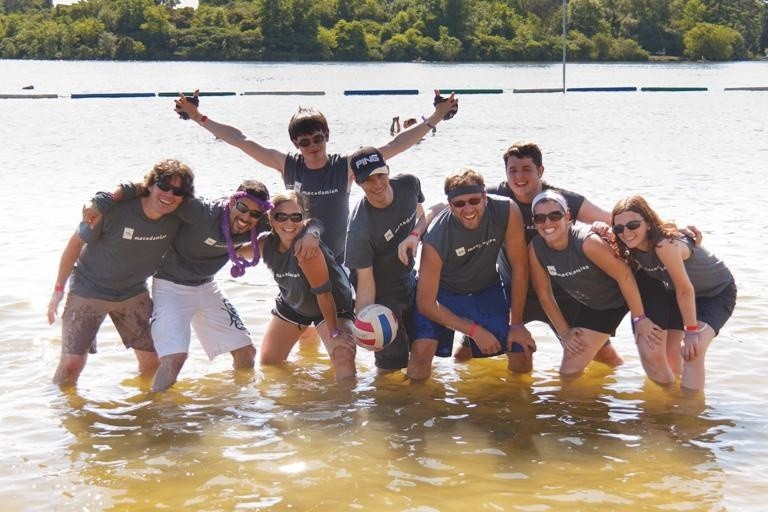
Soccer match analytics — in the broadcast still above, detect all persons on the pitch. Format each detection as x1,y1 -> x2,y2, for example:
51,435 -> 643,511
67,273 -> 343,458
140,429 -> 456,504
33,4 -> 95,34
396,143 -> 702,367
342,144 -> 426,372
80,181 -> 326,394
173,89 -> 459,340
403,170 -> 537,381
523,189 -> 674,388
48,160 -> 195,384
229,189 -> 358,385
591,196 -> 738,392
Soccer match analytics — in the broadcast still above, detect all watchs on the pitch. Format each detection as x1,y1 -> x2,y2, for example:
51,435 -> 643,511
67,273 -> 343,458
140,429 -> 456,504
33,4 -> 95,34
304,228 -> 321,241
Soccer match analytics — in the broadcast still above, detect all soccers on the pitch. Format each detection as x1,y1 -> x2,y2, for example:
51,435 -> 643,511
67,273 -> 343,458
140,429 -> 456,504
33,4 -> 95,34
352,303 -> 399,350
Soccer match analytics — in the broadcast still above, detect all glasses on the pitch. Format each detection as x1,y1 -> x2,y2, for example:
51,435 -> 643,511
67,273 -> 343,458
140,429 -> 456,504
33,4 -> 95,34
156,180 -> 185,196
612,218 -> 646,233
452,194 -> 484,206
533,212 -> 565,224
274,213 -> 303,222
298,134 -> 326,146
236,200 -> 263,217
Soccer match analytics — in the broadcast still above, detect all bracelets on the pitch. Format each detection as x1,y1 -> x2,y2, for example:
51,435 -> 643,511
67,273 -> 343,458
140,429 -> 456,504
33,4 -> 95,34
55,285 -> 64,292
199,115 -> 208,126
510,322 -> 524,331
466,321 -> 478,337
632,314 -> 647,324
408,231 -> 422,241
421,116 -> 434,129
328,327 -> 344,338
683,325 -> 698,332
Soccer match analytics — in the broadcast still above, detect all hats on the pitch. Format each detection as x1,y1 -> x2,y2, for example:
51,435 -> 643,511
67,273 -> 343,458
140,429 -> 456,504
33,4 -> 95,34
350,146 -> 389,181
